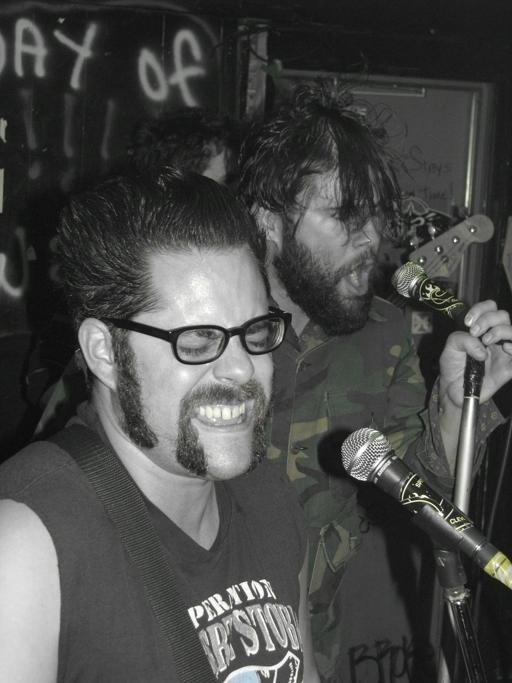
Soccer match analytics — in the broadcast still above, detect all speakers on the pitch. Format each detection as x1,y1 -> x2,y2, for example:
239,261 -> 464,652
266,67 -> 503,314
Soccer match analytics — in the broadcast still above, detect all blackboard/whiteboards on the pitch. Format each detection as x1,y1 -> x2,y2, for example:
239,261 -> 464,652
0,2 -> 242,459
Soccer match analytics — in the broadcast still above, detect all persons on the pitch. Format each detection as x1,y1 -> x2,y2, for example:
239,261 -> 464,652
0,163 -> 323,682
230,76 -> 511,683
29,104 -> 258,442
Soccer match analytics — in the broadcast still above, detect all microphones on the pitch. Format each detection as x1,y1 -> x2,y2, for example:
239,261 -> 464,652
389,260 -> 504,346
340,426 -> 512,592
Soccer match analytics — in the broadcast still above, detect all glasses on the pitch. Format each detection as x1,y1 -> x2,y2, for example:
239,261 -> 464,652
102,303 -> 293,366
